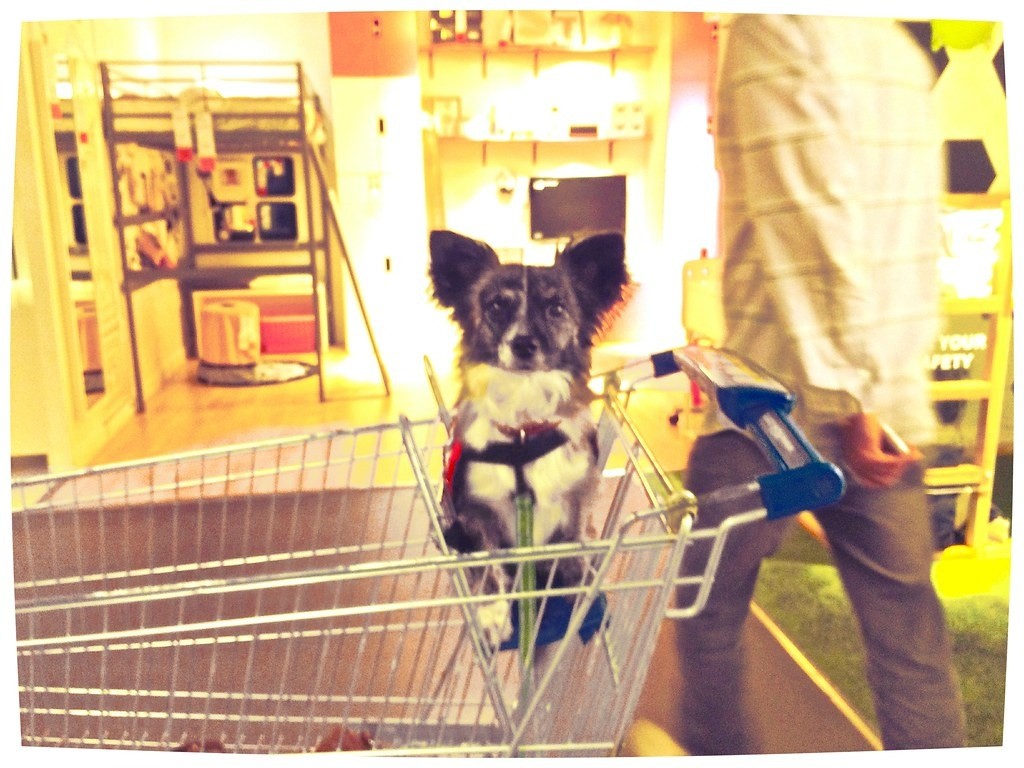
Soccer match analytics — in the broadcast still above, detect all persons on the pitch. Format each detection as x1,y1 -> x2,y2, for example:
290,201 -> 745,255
673,11 -> 968,757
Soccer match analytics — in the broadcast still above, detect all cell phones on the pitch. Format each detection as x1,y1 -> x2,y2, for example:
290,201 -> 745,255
879,422 -> 910,456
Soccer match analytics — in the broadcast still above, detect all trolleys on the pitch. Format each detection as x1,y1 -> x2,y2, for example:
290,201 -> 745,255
12,342 -> 852,758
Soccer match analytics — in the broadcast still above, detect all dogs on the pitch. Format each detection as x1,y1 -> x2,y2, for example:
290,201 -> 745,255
426,224 -> 631,661
